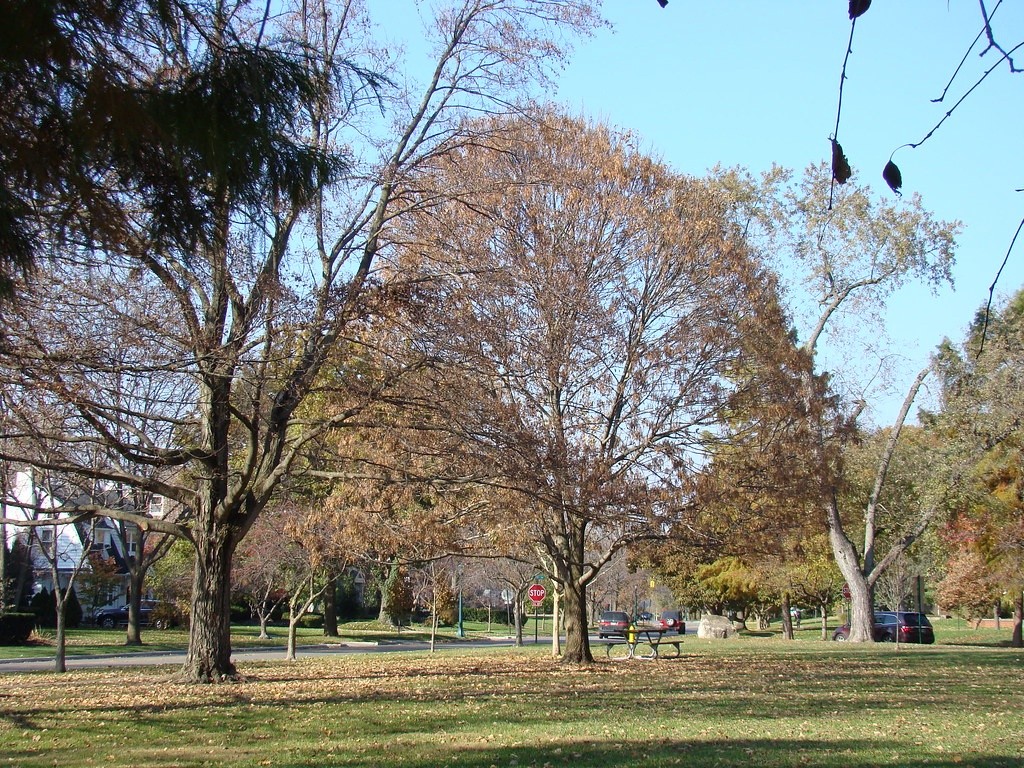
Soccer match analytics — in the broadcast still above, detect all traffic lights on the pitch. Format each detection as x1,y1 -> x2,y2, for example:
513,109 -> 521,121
650,579 -> 656,588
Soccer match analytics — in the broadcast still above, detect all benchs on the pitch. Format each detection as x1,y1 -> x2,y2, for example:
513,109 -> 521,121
642,640 -> 684,646
603,642 -> 643,645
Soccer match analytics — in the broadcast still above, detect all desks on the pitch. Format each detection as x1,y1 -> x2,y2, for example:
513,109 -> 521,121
606,629 -> 680,661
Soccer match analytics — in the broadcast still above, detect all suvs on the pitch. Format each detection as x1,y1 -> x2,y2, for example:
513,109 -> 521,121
659,610 -> 685,634
95,600 -> 184,630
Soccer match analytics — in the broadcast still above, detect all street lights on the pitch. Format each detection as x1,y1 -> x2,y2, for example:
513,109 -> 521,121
634,584 -> 638,625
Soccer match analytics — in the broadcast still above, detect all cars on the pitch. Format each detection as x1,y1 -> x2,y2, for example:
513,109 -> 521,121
642,614 -> 650,620
831,611 -> 935,644
598,611 -> 635,639
790,608 -> 799,616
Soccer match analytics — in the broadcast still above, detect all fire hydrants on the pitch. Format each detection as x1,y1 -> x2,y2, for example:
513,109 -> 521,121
629,626 -> 635,644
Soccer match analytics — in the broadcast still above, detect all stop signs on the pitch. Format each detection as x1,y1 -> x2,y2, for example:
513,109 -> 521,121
842,582 -> 852,599
528,584 -> 546,602
